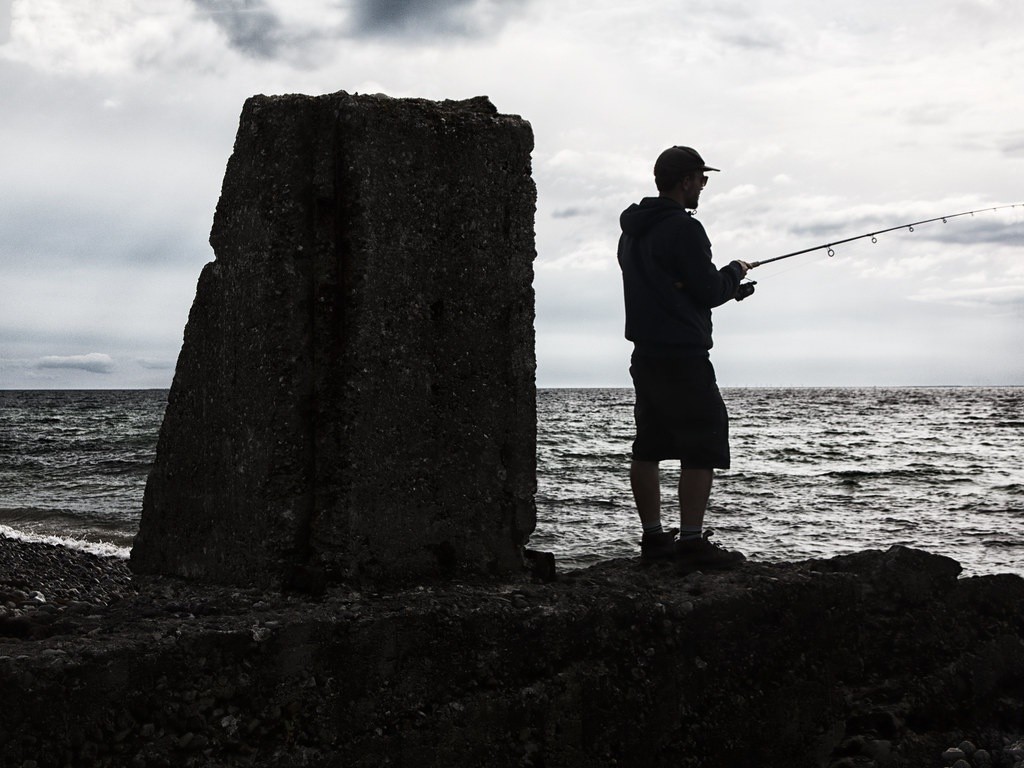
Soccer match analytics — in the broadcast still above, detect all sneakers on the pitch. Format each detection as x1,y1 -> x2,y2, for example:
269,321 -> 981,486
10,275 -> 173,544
673,530 -> 746,569
638,526 -> 680,561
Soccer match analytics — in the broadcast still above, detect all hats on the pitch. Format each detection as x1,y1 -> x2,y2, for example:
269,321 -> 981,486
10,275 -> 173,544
654,146 -> 720,175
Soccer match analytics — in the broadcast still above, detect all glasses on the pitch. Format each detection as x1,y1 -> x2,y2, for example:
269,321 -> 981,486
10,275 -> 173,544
687,175 -> 708,187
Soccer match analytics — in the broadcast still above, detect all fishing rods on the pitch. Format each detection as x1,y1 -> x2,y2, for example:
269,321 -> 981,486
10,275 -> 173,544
732,203 -> 1024,302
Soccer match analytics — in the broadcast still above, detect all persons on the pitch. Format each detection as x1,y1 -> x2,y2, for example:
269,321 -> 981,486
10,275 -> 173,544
617,145 -> 757,577
